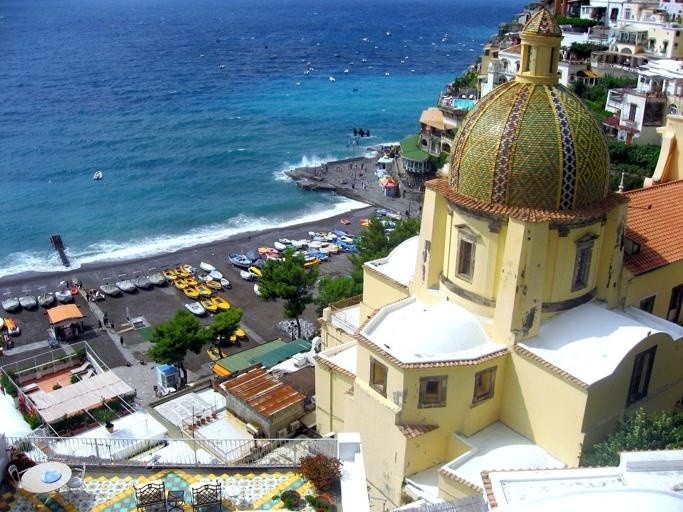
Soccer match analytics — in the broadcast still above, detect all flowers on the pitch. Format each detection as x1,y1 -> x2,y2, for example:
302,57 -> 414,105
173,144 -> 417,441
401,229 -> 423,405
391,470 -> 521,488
297,453 -> 343,488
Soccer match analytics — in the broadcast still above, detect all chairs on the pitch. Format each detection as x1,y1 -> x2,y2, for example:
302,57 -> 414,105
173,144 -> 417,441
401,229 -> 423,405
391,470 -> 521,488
80,369 -> 93,380
190,480 -> 222,512
59,463 -> 86,501
70,361 -> 90,373
133,482 -> 167,512
8,464 -> 37,504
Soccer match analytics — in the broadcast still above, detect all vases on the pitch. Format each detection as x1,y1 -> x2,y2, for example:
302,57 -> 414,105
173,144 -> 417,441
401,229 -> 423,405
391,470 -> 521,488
320,484 -> 333,491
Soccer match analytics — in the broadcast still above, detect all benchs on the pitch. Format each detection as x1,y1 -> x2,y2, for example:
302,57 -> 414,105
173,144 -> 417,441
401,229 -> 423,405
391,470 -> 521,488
21,383 -> 37,393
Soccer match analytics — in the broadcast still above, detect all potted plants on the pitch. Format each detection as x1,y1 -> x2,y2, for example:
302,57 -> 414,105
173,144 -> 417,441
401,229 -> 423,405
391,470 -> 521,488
305,493 -> 333,512
272,491 -> 301,509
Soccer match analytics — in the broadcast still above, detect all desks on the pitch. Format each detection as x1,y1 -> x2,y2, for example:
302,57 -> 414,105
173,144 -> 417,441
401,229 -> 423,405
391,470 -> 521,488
166,491 -> 185,512
21,461 -> 72,507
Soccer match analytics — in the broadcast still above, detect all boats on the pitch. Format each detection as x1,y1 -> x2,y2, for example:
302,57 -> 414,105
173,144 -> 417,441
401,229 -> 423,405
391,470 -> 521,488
2,280 -> 75,312
8,324 -> 20,337
163,261 -> 246,344
94,170 -> 102,179
341,218 -> 351,224
87,269 -> 167,301
361,208 -> 405,233
228,228 -> 360,296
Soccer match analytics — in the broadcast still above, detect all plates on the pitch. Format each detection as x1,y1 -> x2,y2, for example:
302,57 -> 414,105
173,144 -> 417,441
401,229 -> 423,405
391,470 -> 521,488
41,471 -> 62,483
